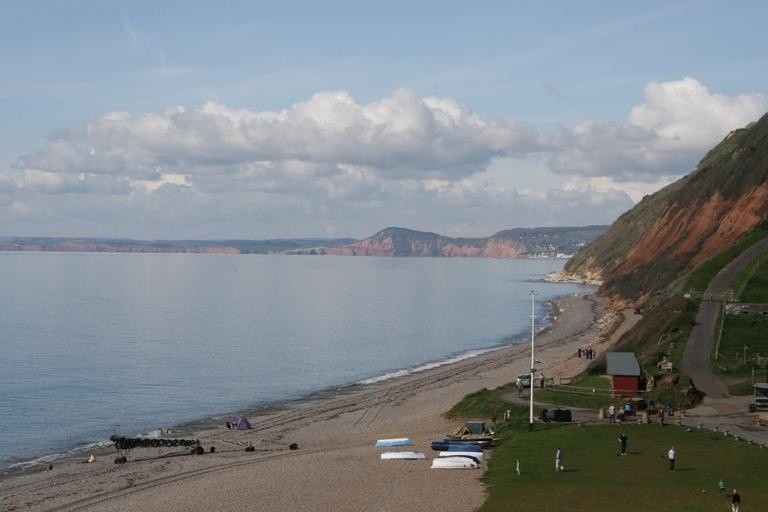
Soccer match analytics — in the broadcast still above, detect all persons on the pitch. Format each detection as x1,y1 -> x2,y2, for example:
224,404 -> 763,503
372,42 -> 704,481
578,347 -> 596,359
608,405 -> 616,423
539,372 -> 545,388
731,489 -> 740,512
719,479 -> 726,497
555,447 -> 566,473
518,381 -> 524,398
618,407 -> 625,422
668,447 -> 676,472
618,433 -> 628,456
659,408 -> 664,425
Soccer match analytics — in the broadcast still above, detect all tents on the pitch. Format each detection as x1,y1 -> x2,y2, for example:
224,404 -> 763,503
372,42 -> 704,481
237,417 -> 251,430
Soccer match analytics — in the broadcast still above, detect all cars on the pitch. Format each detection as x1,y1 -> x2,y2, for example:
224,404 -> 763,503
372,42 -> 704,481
514,374 -> 533,388
544,407 -> 571,424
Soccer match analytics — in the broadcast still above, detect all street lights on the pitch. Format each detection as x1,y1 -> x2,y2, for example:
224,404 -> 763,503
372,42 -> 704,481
528,288 -> 538,430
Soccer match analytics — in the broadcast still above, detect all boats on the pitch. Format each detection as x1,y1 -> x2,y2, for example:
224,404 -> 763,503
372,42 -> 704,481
374,435 -> 493,470
